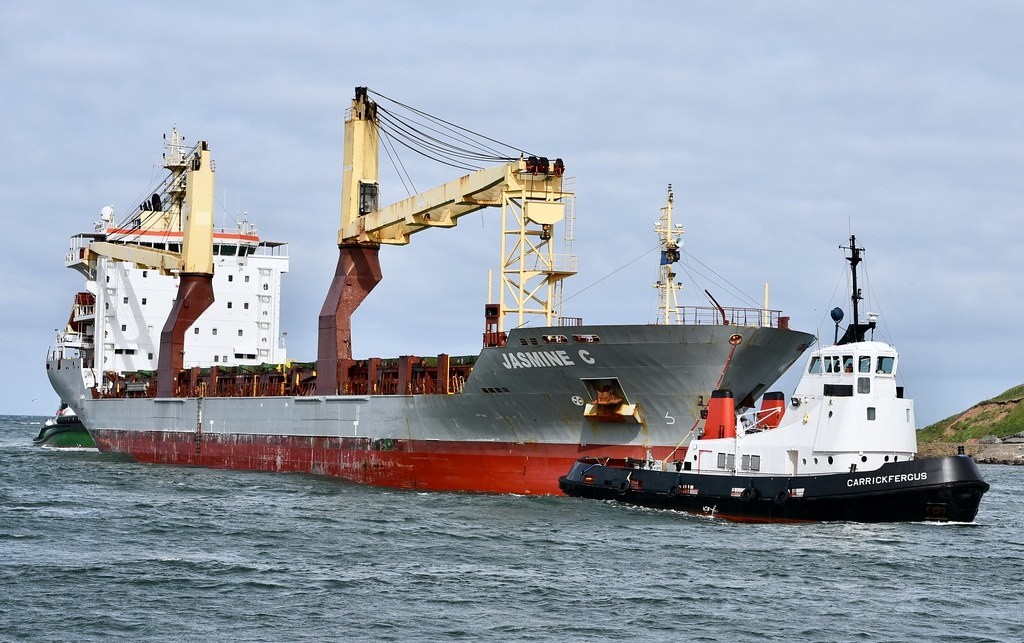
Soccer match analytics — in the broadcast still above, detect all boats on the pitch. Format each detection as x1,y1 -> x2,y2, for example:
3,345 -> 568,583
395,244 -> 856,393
556,236 -> 991,526
31,398 -> 97,449
47,83 -> 817,494
